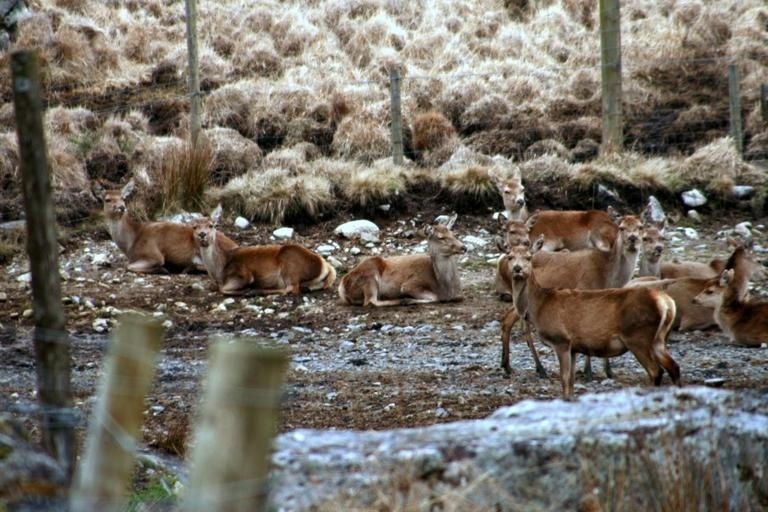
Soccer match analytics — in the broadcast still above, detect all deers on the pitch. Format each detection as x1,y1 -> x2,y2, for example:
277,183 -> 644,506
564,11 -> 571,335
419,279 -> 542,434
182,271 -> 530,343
637,216 -> 671,276
690,268 -> 768,354
337,212 -> 468,308
487,166 -> 610,248
180,203 -> 336,297
494,232 -> 681,396
499,209 -> 540,251
624,231 -> 768,332
499,205 -> 650,382
91,175 -> 240,273
